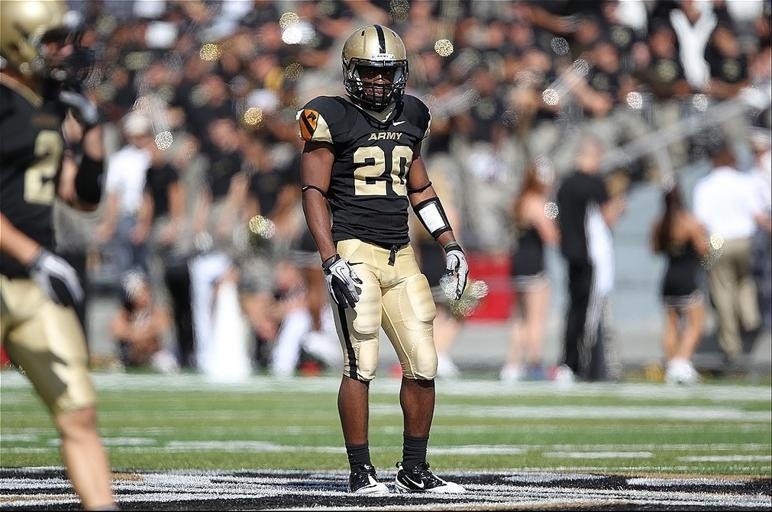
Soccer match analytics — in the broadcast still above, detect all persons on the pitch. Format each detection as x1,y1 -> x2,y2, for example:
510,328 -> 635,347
296,25 -> 468,496
2,0 -> 771,386
0,0 -> 124,512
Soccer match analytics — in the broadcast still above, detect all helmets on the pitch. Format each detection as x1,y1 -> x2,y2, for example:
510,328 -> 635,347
341,25 -> 408,108
0,1 -> 73,82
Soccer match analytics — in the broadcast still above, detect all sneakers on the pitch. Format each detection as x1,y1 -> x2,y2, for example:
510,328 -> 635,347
347,465 -> 390,497
395,462 -> 467,496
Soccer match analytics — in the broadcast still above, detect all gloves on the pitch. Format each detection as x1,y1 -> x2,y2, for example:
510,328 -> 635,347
320,253 -> 363,310
443,242 -> 470,301
30,250 -> 87,306
